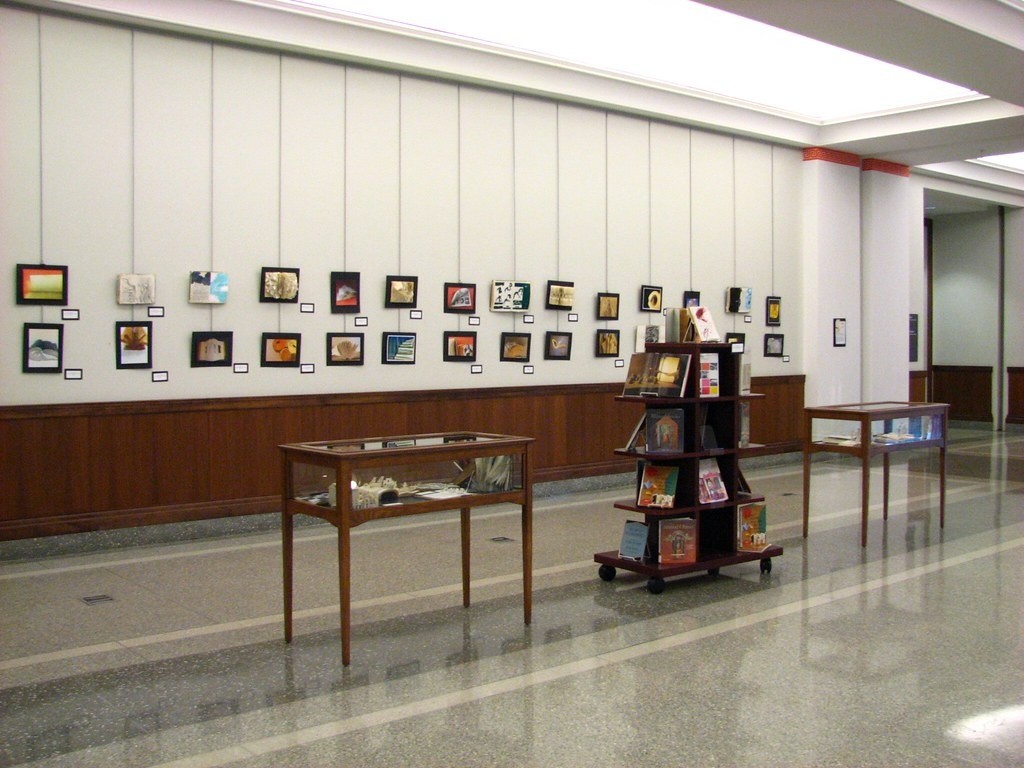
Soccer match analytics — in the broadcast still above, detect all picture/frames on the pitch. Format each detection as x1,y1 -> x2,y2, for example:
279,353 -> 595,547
189,325 -> 662,369
833,318 -> 846,347
116,267 -> 420,314
764,334 -> 784,357
16,263 -> 69,307
22,323 -> 63,374
116,320 -> 152,370
683,291 -> 700,309
765,296 -> 781,327
443,280 -> 619,320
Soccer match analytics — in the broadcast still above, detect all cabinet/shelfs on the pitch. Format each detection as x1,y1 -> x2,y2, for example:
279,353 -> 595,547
594,341 -> 784,594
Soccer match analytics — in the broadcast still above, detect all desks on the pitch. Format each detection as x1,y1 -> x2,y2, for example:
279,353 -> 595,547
276,432 -> 537,666
799,399 -> 951,548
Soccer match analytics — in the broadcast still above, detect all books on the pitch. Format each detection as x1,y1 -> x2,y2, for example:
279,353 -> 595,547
637,465 -> 679,508
700,353 -> 719,398
737,502 -> 767,548
666,307 -> 696,342
646,409 -> 684,453
698,457 -> 729,504
658,517 -> 696,563
623,352 -> 692,397
